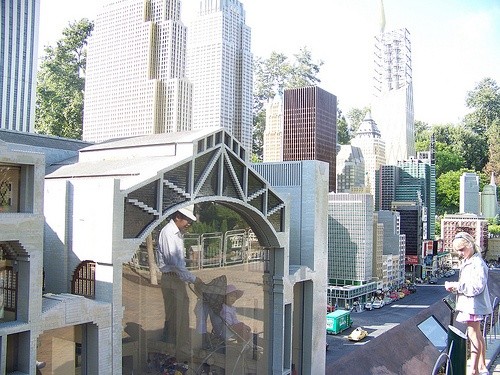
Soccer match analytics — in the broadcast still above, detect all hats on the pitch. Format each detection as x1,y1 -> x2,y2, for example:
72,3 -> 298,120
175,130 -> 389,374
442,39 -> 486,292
178,203 -> 197,222
225,285 -> 244,300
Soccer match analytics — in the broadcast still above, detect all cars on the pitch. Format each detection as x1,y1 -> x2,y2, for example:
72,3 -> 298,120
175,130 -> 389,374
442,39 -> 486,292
365,288 -> 416,311
429,278 -> 437,284
444,270 -> 455,277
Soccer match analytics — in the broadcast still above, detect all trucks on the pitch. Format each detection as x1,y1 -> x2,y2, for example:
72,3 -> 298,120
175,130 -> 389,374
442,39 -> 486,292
326,309 -> 353,335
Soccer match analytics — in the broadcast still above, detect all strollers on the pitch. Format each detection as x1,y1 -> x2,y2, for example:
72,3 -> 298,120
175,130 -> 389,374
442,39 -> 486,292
186,275 -> 263,360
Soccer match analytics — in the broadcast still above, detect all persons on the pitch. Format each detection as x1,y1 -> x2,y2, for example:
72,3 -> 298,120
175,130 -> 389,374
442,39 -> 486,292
445,231 -> 493,375
156,204 -> 202,346
219,284 -> 251,344
